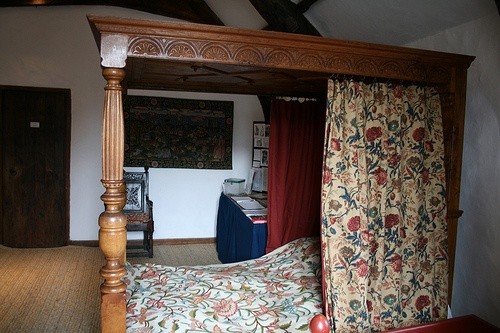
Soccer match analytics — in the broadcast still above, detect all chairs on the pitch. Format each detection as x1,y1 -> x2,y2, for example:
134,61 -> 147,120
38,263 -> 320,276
121,165 -> 154,259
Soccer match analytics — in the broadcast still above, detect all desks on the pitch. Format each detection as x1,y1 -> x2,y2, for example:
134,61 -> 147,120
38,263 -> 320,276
217,191 -> 270,264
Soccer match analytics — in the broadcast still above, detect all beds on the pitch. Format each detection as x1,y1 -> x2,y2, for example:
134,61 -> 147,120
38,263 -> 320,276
124,235 -> 324,333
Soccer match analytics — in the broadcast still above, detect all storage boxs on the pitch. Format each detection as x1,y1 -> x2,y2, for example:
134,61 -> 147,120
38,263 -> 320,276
223,177 -> 246,196
